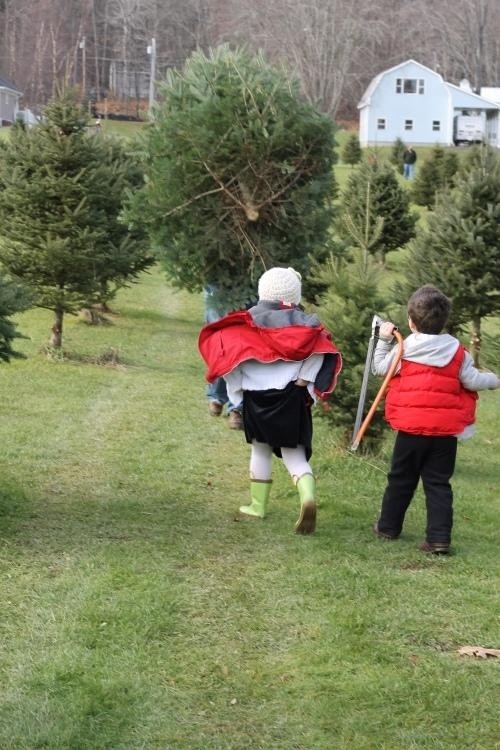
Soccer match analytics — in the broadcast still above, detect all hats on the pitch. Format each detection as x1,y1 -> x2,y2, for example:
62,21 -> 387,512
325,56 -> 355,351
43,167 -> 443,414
257,267 -> 302,306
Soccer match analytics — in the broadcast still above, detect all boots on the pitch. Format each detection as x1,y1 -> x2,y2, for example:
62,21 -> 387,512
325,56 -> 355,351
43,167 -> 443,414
295,473 -> 317,536
239,478 -> 273,519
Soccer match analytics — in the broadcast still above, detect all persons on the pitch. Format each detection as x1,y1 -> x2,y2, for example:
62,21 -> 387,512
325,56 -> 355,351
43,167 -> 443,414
403,144 -> 416,180
205,275 -> 243,428
195,266 -> 345,535
370,286 -> 500,555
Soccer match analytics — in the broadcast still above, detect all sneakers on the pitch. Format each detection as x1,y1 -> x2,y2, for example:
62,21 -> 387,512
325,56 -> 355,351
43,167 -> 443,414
419,540 -> 450,553
229,409 -> 244,430
373,523 -> 399,540
209,400 -> 221,416
